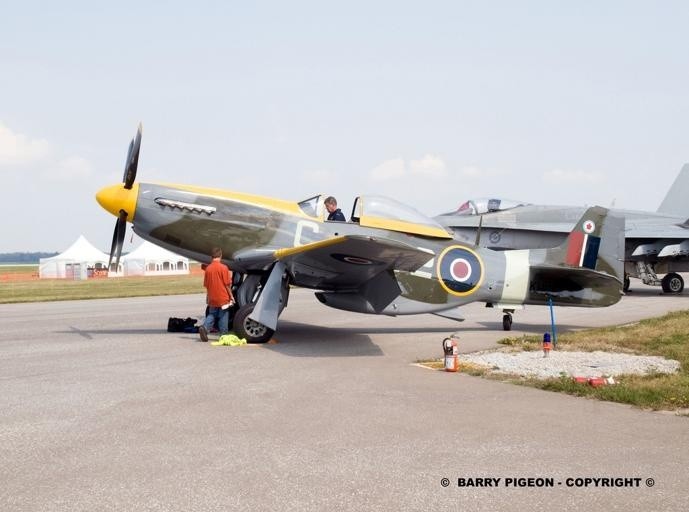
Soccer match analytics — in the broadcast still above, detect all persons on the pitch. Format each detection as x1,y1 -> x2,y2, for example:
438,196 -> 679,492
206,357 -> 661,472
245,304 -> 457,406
324,195 -> 346,222
198,246 -> 237,342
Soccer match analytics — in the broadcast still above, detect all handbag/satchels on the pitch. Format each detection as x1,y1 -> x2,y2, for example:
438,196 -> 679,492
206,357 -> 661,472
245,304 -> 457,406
168,318 -> 185,332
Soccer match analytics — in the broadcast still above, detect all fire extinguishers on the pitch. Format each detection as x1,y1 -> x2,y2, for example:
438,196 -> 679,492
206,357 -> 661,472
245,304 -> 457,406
443,332 -> 460,372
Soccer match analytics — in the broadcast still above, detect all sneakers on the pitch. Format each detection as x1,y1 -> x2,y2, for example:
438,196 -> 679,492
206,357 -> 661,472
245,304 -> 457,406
199,326 -> 208,342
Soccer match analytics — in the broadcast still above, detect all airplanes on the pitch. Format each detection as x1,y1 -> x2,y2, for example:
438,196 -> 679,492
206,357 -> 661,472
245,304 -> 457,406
95,122 -> 688,343
